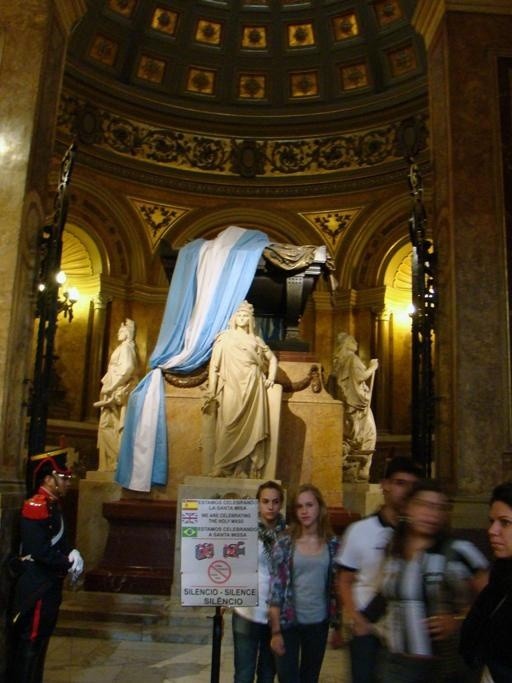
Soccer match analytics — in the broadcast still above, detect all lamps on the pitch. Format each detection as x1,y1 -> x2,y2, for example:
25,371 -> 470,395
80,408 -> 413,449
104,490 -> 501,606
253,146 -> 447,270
37,270 -> 80,322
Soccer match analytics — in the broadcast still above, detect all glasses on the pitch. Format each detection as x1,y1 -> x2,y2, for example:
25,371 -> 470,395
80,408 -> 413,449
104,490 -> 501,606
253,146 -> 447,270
55,473 -> 72,480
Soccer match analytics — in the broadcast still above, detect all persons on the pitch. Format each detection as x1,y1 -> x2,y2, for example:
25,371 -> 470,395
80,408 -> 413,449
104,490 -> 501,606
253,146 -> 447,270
265,483 -> 344,682
233,481 -> 290,682
208,300 -> 278,479
333,332 -> 379,475
6,475 -> 84,683
330,457 -> 512,683
97,318 -> 139,472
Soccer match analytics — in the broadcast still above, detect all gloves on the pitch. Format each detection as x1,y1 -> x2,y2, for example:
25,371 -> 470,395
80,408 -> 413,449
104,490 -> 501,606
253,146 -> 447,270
68,548 -> 84,582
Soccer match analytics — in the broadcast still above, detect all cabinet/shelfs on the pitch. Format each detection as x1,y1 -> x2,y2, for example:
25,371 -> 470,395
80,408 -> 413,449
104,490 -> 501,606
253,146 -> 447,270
86,497 -> 361,596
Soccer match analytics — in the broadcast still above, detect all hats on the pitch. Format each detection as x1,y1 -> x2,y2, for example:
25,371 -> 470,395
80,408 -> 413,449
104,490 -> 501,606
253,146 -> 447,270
385,456 -> 426,477
30,447 -> 74,479
